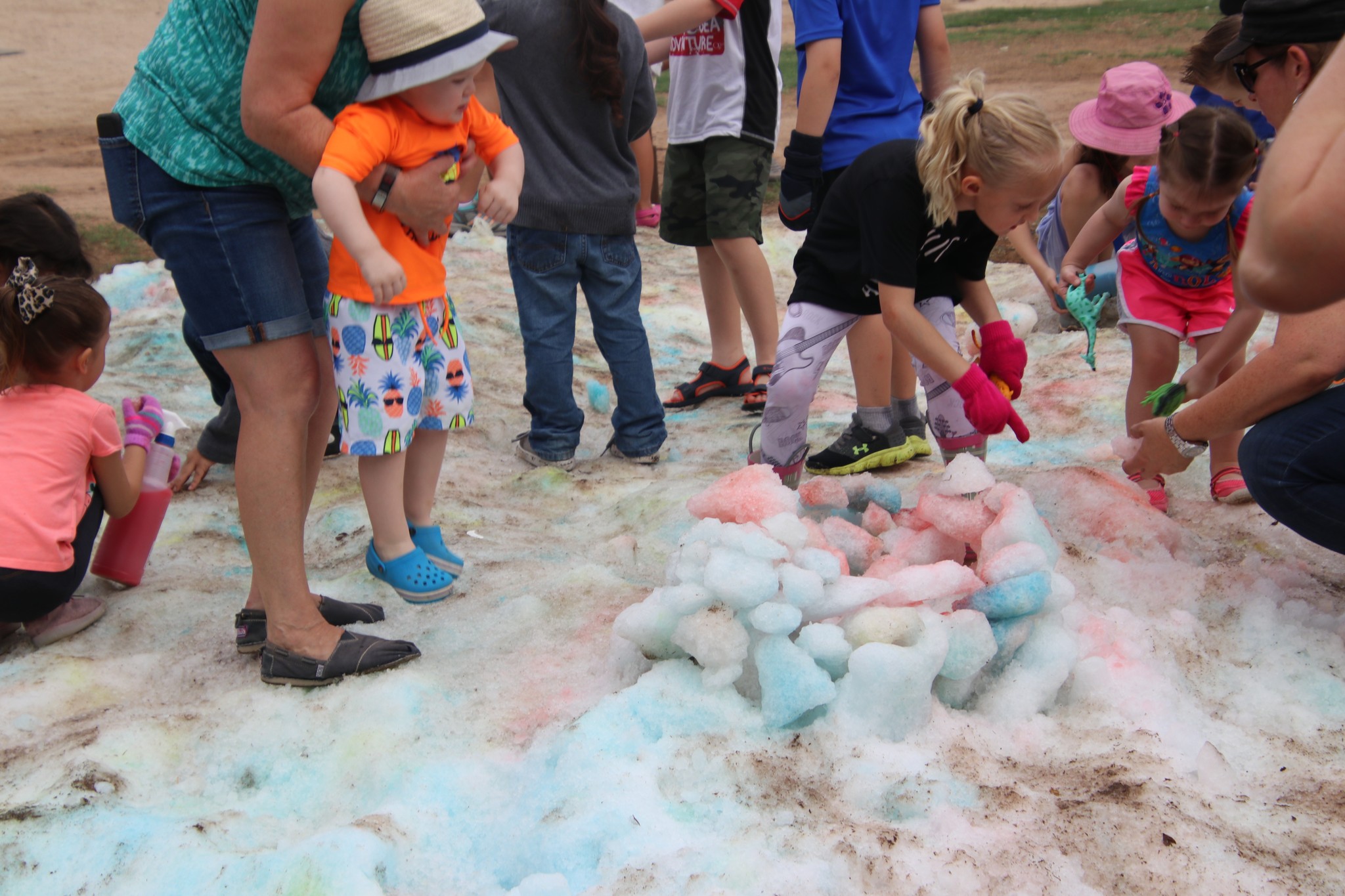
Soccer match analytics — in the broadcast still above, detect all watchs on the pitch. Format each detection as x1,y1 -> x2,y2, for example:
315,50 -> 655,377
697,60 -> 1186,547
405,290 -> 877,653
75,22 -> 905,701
1164,410 -> 1208,460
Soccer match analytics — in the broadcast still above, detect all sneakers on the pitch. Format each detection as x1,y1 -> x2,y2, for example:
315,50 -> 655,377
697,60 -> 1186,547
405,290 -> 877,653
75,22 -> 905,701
899,412 -> 931,456
510,429 -> 577,471
448,192 -> 507,236
23,596 -> 105,649
599,436 -> 660,465
804,413 -> 917,475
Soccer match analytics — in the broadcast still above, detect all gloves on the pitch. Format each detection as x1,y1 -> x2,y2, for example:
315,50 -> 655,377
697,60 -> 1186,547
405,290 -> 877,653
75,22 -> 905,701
951,363 -> 1030,443
121,394 -> 164,454
978,320 -> 1028,399
778,130 -> 824,233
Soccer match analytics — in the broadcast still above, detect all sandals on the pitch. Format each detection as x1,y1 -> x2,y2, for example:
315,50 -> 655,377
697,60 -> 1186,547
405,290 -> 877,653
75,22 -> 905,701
741,364 -> 774,412
408,520 -> 463,577
664,356 -> 752,409
366,538 -> 454,603
635,204 -> 661,227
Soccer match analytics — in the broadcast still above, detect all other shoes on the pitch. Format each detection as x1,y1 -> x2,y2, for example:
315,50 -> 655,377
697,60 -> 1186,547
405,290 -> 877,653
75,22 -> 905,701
1209,466 -> 1253,504
233,595 -> 384,651
1127,471 -> 1168,513
260,629 -> 423,685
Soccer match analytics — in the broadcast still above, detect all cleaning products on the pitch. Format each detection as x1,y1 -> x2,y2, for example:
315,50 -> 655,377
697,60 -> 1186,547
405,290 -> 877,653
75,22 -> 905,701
88,400 -> 190,588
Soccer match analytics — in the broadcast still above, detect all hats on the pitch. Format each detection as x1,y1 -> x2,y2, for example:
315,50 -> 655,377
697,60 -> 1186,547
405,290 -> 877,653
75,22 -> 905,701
353,0 -> 519,103
1068,60 -> 1196,155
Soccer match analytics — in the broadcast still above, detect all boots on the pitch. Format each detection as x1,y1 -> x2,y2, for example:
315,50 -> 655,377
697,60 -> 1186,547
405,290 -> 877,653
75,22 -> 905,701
925,409 -> 989,559
746,421 -> 811,490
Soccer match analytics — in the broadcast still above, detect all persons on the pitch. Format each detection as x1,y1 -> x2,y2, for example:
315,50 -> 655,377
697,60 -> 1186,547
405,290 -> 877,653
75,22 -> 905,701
0,0 -> 1343,692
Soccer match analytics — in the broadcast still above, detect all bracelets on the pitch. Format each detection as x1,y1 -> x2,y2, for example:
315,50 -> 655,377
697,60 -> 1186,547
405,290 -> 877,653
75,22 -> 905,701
370,164 -> 399,211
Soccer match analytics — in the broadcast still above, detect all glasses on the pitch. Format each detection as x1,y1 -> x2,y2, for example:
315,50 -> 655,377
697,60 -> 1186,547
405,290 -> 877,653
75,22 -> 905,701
1234,53 -> 1285,92
